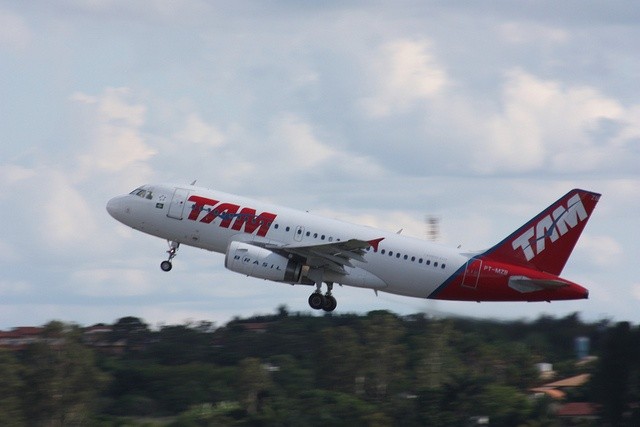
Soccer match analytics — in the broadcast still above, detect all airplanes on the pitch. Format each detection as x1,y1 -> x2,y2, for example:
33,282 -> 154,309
104,180 -> 603,313
